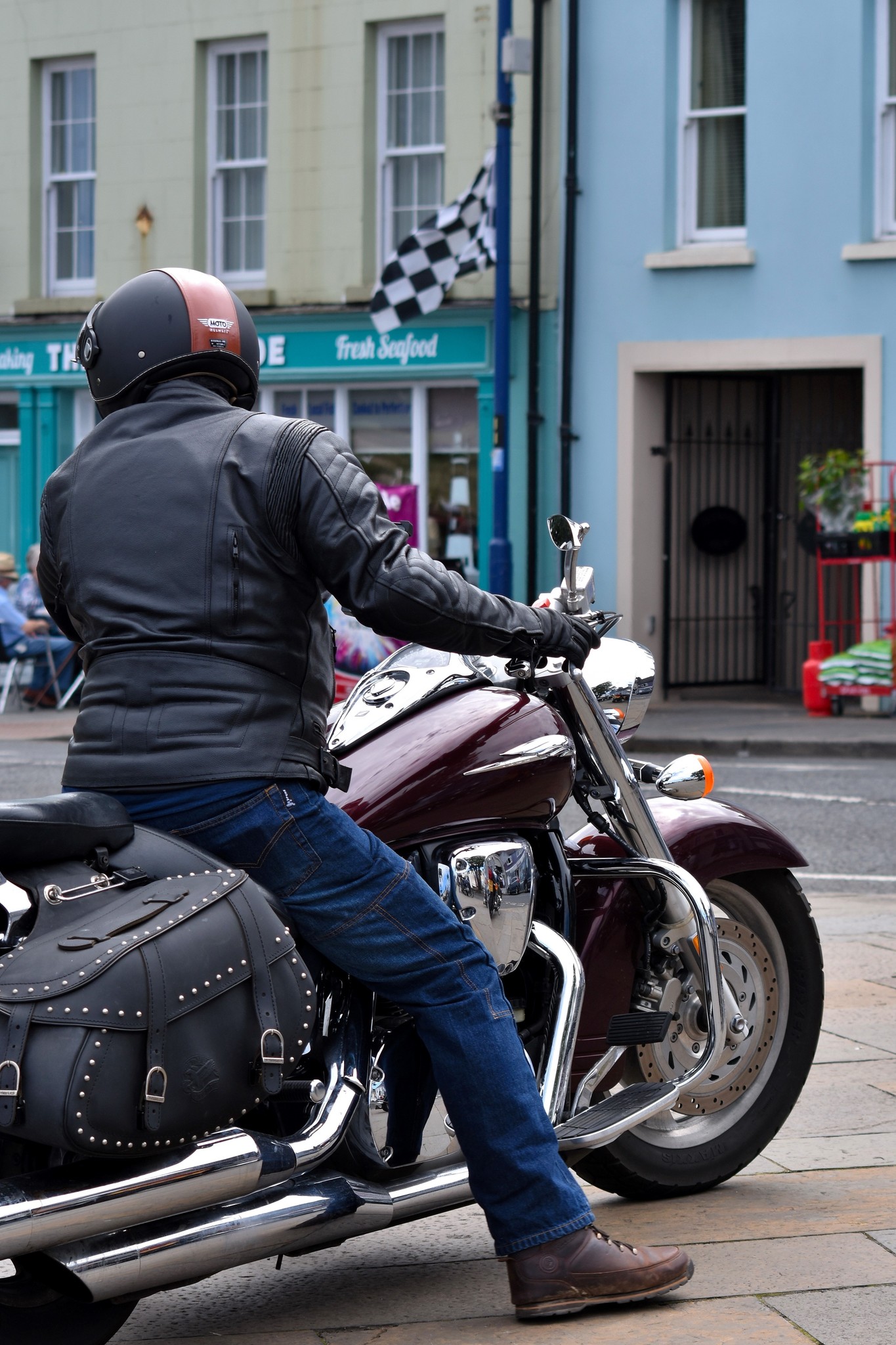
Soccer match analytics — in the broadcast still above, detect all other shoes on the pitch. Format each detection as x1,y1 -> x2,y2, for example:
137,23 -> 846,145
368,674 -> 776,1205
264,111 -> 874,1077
38,694 -> 56,708
22,688 -> 39,703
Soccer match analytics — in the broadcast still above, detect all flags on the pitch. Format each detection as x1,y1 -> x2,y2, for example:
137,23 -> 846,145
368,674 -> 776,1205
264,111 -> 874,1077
370,153 -> 498,336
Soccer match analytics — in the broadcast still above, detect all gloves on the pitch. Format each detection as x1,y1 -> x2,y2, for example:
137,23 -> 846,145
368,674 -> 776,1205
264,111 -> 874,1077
533,607 -> 601,672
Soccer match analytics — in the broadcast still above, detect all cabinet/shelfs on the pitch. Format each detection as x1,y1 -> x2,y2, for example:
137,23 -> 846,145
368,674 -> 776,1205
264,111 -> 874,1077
814,461 -> 896,695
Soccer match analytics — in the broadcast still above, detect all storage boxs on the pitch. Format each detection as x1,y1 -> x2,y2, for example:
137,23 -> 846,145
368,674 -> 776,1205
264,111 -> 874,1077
820,531 -> 889,557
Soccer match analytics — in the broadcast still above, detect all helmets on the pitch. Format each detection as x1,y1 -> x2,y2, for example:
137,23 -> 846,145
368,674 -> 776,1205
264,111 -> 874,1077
71,267 -> 260,420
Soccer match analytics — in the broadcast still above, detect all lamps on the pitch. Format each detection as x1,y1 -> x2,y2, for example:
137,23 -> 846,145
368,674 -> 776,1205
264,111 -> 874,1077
136,207 -> 154,236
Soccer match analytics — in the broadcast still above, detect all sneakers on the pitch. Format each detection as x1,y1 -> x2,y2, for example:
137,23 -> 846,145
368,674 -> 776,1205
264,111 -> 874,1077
497,1224 -> 694,1321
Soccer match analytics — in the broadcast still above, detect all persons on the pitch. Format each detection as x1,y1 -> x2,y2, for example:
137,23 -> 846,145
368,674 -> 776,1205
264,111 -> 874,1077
460,865 -> 503,909
0,548 -> 80,709
34,268 -> 695,1319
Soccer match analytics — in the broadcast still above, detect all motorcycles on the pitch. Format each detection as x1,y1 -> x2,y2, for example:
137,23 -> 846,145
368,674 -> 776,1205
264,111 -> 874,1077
0,511 -> 827,1344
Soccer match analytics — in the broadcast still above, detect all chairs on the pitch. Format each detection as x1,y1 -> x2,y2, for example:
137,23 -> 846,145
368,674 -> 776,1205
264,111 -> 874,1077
0,625 -> 62,714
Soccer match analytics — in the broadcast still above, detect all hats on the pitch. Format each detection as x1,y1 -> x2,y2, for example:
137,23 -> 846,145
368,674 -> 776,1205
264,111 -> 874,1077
0,553 -> 19,580
